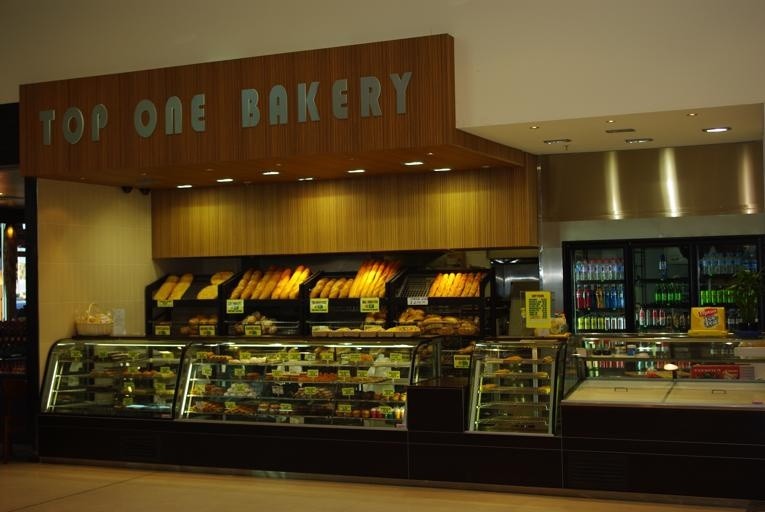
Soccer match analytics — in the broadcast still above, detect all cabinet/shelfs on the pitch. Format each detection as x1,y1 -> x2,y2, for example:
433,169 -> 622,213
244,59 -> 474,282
557,331 -> 764,507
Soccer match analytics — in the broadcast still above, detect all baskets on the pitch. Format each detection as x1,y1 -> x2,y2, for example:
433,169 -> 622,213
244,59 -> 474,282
75,303 -> 114,337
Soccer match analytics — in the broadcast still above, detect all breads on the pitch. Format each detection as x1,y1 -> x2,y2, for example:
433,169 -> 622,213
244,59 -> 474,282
180,314 -> 218,335
348,257 -> 403,299
399,308 -> 475,336
234,312 -> 278,334
168,273 -> 193,299
210,271 -> 235,285
198,285 -> 217,298
154,275 -> 179,300
230,264 -> 309,300
310,277 -> 354,300
427,272 -> 487,297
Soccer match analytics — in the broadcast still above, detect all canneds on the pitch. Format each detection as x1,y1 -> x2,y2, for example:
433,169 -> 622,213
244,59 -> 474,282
700,289 -> 736,305
578,313 -> 626,329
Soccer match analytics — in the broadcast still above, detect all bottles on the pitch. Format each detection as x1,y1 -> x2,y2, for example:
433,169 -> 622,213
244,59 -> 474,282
577,337 -> 691,380
699,249 -> 761,330
572,257 -> 628,331
638,254 -> 691,331
